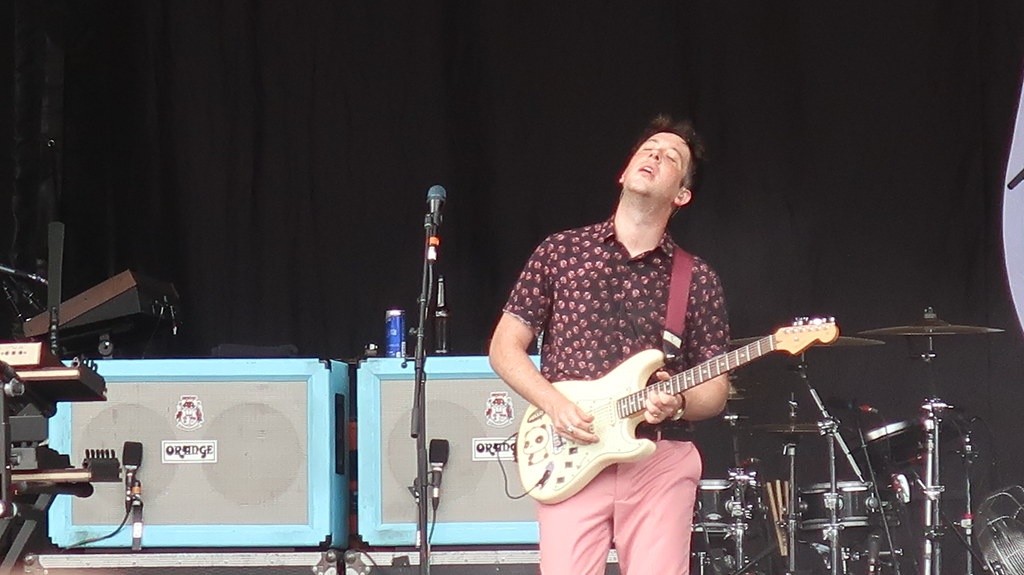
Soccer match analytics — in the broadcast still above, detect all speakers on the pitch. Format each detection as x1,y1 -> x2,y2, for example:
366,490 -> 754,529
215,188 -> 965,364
357,355 -> 539,544
47,358 -> 349,549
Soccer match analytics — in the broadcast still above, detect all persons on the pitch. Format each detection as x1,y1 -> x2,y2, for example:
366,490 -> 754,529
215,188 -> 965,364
489,114 -> 733,575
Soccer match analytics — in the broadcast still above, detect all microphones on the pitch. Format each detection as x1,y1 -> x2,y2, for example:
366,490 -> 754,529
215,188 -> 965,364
122,441 -> 143,512
848,404 -> 879,415
429,438 -> 449,507
427,184 -> 447,260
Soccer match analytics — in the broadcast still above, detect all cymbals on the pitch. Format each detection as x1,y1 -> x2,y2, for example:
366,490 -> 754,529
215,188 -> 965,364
857,322 -> 1006,337
729,334 -> 886,348
744,422 -> 822,434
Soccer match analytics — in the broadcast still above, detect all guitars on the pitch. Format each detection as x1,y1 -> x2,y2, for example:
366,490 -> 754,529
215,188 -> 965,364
515,315 -> 839,507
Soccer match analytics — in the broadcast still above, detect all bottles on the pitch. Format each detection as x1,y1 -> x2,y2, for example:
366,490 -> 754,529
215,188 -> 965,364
434,274 -> 449,355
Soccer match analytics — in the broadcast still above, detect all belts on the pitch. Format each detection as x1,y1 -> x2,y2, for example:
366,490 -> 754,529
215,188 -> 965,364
638,427 -> 692,442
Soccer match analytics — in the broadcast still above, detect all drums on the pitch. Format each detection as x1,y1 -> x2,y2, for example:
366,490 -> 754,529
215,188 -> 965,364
799,484 -> 876,527
693,479 -> 766,535
864,419 -> 958,468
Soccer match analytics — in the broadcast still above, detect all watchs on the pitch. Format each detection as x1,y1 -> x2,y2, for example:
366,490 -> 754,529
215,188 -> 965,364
669,392 -> 687,422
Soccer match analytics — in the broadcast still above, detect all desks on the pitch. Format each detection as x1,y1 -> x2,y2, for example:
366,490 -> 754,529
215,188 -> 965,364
47,358 -> 352,552
356,357 -> 542,546
